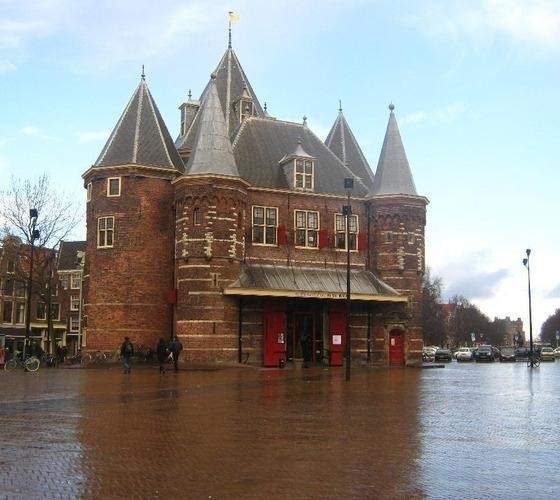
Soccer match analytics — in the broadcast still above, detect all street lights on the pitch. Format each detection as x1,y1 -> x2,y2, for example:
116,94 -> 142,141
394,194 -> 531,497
523,250 -> 533,355
341,176 -> 353,382
26,208 -> 39,339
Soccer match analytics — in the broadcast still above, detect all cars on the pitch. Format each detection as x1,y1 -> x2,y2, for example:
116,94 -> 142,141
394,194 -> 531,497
539,346 -> 560,361
435,349 -> 452,362
516,347 -> 540,358
499,348 -> 516,362
492,347 -> 501,358
453,348 -> 473,362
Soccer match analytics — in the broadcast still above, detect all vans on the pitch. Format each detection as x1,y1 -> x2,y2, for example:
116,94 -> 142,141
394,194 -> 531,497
422,345 -> 440,362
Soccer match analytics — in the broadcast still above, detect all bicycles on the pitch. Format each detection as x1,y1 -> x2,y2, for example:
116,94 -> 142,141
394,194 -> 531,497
4,350 -> 40,373
69,351 -> 106,368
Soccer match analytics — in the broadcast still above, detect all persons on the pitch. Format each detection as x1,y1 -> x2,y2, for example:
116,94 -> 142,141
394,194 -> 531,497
168,336 -> 183,372
156,338 -> 168,373
120,336 -> 134,374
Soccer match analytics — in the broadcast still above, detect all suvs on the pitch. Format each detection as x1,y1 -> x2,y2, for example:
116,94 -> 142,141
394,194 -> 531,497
472,345 -> 495,362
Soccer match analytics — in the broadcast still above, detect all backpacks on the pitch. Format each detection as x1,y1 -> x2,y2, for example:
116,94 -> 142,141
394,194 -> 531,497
124,341 -> 133,357
